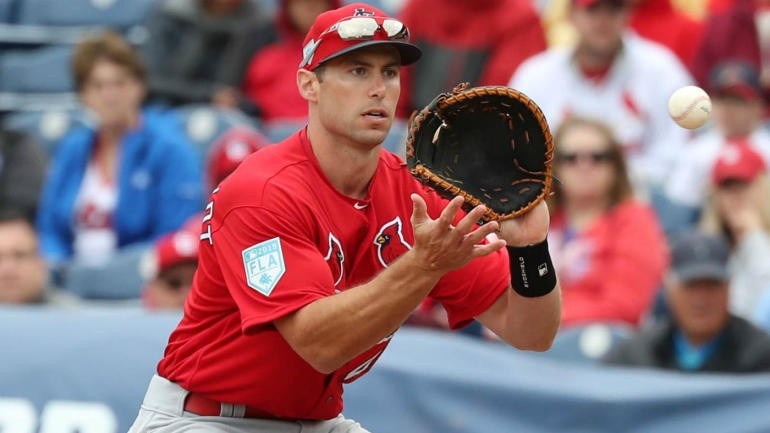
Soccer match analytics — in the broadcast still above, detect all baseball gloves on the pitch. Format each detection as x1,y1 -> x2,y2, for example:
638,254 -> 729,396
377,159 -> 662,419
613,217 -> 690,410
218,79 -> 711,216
405,82 -> 555,228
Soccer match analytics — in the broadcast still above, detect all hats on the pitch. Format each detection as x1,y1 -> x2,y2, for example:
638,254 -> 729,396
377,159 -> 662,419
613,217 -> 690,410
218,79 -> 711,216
713,145 -> 766,187
664,231 -> 730,286
139,230 -> 201,284
300,4 -> 421,70
705,60 -> 763,103
204,129 -> 276,190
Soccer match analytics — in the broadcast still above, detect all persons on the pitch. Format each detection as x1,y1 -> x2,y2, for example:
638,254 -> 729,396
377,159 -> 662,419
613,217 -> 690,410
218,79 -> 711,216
663,58 -> 770,204
546,110 -> 671,334
500,0 -> 709,175
134,227 -> 205,311
34,27 -> 214,301
624,1 -> 759,111
240,0 -> 411,123
123,2 -> 566,432
128,0 -> 272,121
699,143 -> 770,334
0,210 -> 82,308
187,124 -> 272,236
599,233 -> 770,375
381,1 -> 549,122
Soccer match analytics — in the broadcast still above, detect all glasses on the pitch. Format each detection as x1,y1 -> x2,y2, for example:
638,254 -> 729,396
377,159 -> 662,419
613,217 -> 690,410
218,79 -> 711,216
300,16 -> 411,69
552,148 -> 617,165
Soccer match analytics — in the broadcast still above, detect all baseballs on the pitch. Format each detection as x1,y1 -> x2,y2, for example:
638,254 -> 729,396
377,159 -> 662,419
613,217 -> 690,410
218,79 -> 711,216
668,85 -> 712,129
